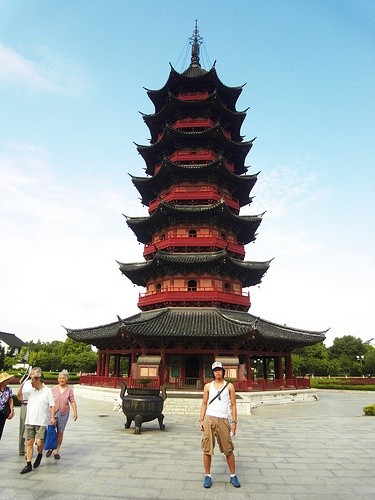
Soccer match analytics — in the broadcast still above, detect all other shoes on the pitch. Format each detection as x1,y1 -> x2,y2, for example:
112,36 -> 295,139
46,449 -> 60,459
20,457 -> 41,474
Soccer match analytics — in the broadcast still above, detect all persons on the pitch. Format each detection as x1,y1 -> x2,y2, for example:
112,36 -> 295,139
200,361 -> 242,489
18,371 -> 56,475
15,366 -> 47,457
46,371 -> 79,459
0,372 -> 16,439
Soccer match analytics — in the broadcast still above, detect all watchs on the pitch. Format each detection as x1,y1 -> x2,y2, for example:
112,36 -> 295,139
232,420 -> 237,424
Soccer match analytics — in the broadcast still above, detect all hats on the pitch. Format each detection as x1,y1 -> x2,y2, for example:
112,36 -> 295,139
0,371 -> 15,384
211,362 -> 225,373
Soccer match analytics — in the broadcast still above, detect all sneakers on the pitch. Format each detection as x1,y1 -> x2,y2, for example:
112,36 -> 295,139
203,475 -> 212,488
229,475 -> 241,488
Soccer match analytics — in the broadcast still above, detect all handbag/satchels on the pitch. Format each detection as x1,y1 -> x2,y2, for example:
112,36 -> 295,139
44,425 -> 58,450
